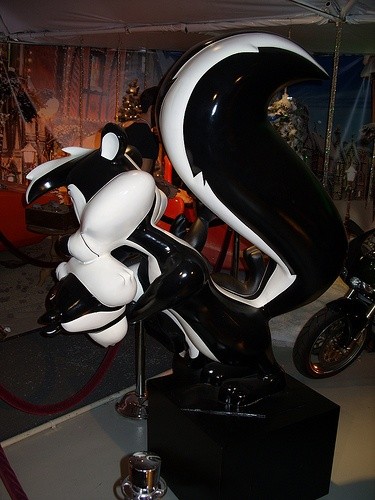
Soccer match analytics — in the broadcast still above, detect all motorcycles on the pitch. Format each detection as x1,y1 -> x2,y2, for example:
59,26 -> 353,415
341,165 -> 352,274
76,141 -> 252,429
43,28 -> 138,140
291,215 -> 375,381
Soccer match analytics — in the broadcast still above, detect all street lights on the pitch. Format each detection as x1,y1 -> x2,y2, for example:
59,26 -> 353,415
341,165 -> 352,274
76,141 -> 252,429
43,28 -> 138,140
344,162 -> 357,217
21,141 -> 37,191
